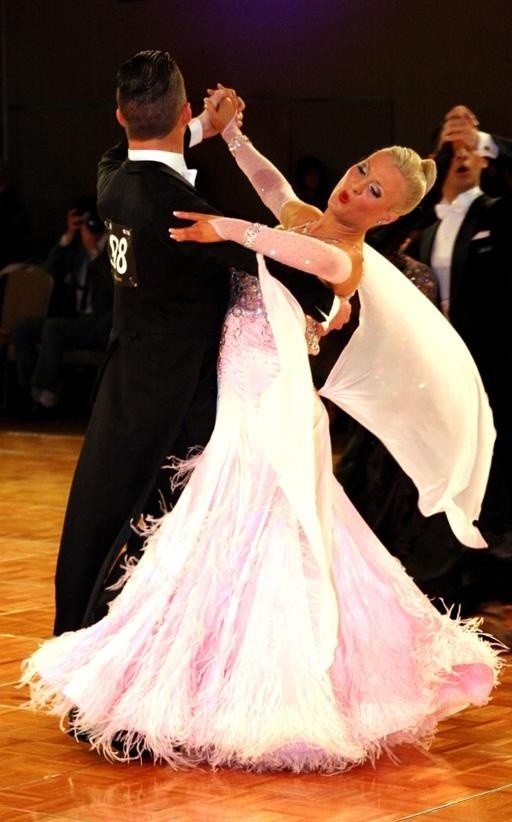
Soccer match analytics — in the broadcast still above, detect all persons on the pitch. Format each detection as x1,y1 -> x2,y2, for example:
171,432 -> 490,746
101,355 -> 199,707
13,83 -> 511,774
330,104 -> 511,619
397,118 -> 510,541
0,155 -> 492,440
51,48 -> 353,767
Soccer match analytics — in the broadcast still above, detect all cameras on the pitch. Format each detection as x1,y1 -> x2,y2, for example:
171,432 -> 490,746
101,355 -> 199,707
75,209 -> 104,236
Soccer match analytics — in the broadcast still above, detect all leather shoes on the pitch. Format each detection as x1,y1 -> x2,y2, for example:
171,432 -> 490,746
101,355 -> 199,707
18,394 -> 61,423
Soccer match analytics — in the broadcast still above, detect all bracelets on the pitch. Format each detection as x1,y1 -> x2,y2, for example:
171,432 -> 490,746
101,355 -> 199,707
227,134 -> 251,153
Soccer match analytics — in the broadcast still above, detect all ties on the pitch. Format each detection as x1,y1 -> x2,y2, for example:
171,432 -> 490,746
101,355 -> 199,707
77,259 -> 94,317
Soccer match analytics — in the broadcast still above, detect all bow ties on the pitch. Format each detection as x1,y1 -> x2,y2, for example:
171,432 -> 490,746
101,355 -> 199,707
434,200 -> 468,223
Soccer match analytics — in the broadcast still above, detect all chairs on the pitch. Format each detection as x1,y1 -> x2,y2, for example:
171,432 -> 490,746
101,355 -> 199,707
1,261 -> 54,408
